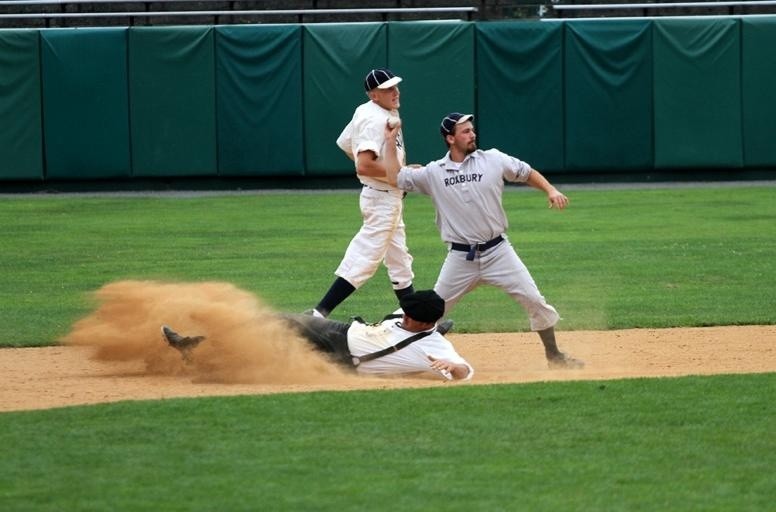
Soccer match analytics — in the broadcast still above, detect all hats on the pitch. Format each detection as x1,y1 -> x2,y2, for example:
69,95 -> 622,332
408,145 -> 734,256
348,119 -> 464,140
400,290 -> 445,323
440,111 -> 474,133
364,67 -> 403,91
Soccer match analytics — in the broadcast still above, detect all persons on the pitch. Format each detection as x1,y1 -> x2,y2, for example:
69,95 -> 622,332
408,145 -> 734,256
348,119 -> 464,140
161,285 -> 476,385
380,107 -> 586,370
302,65 -> 453,337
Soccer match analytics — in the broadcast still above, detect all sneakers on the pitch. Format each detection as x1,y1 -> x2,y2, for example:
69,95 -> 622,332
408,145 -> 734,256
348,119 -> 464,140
160,326 -> 195,363
547,352 -> 584,369
437,319 -> 452,334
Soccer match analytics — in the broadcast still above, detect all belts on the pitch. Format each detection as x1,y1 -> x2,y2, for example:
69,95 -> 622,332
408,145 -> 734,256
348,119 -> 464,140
452,235 -> 503,251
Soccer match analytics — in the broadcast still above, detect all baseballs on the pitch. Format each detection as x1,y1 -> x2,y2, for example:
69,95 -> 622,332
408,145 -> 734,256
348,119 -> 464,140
388,117 -> 401,128
